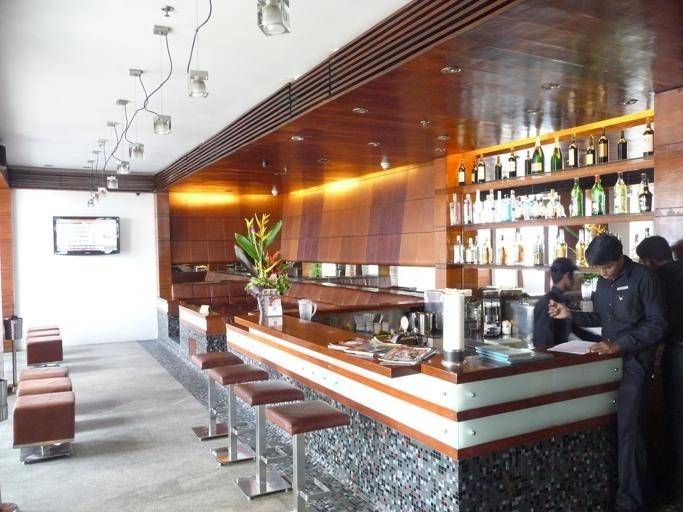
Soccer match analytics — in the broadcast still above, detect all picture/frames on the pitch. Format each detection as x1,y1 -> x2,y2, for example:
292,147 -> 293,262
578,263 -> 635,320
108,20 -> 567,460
52,215 -> 119,255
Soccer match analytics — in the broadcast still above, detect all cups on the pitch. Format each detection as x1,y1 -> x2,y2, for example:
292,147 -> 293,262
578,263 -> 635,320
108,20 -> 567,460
297,298 -> 317,320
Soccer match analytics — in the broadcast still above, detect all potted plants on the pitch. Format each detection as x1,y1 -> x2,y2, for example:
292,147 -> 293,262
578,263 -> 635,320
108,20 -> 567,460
234,211 -> 295,318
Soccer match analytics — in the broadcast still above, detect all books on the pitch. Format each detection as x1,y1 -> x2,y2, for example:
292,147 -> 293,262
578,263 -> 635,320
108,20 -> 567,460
475,336 -> 534,366
328,329 -> 437,366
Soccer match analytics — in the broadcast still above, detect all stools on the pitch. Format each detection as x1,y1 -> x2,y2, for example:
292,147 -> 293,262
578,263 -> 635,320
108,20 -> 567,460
266,399 -> 352,512
190,352 -> 244,442
232,378 -> 304,500
12,327 -> 75,464
207,361 -> 269,465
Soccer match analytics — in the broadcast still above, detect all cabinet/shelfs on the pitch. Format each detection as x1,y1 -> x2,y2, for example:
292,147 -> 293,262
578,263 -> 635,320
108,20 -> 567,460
449,107 -> 655,303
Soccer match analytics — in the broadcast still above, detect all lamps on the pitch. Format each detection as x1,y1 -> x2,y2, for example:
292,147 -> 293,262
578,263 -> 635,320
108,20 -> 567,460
143,24 -> 173,135
123,68 -> 147,162
271,187 -> 278,197
84,98 -> 129,205
186,0 -> 213,99
257,0 -> 290,37
380,156 -> 391,170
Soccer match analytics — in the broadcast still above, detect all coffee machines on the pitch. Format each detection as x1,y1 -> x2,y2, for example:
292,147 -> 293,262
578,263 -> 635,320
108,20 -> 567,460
478,286 -> 501,338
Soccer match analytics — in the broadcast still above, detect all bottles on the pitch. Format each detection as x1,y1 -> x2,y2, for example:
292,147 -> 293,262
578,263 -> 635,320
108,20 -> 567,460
447,117 -> 655,268
407,309 -> 435,335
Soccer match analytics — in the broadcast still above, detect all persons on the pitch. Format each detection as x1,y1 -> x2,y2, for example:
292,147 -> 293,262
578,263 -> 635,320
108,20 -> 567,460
547,234 -> 668,512
636,235 -> 683,480
533,257 -> 601,347
